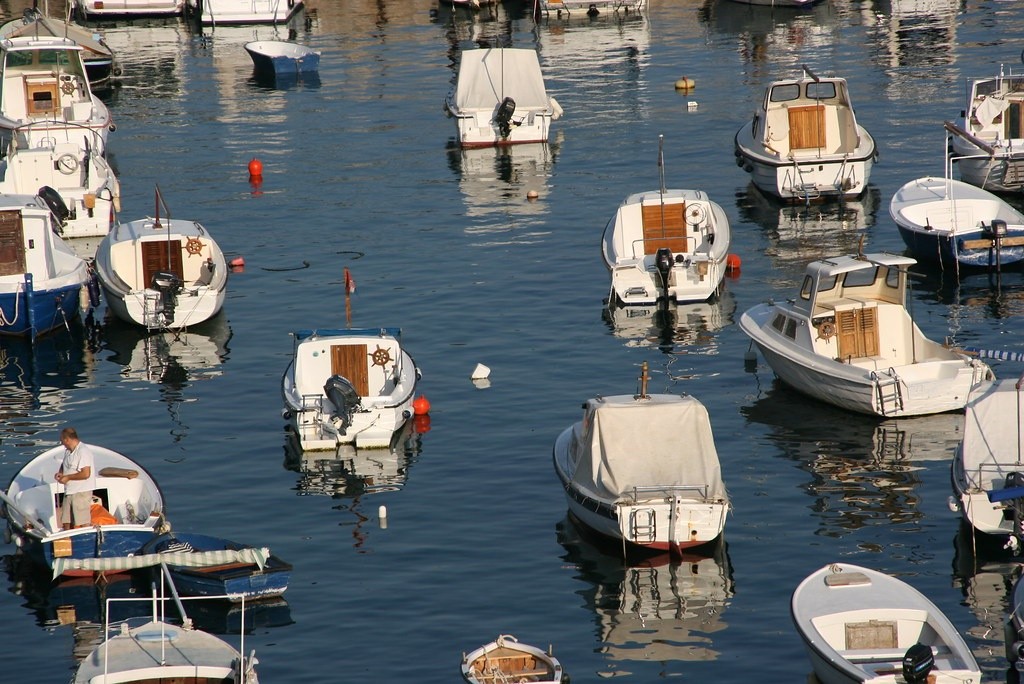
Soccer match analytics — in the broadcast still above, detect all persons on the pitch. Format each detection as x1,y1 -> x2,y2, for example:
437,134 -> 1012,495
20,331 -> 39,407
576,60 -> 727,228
55,427 -> 96,531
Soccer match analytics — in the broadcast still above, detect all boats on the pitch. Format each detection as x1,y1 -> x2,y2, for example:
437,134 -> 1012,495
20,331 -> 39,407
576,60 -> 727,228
187,0 -> 307,27
281,424 -> 424,501
600,275 -> 738,361
93,184 -> 230,337
1,441 -> 166,581
140,531 -> 295,605
789,562 -> 982,684
550,361 -> 731,556
553,505 -> 737,629
443,42 -> 566,148
0,310 -> 100,411
954,375 -> 1024,561
243,39 -> 324,75
279,266 -> 423,454
531,0 -> 649,25
888,174 -> 1024,275
600,135 -> 732,310
739,230 -> 996,421
73,565 -> 261,684
460,634 -> 571,684
943,62 -> 1024,198
458,144 -> 553,187
740,372 -> 967,483
98,304 -> 236,450
734,63 -> 880,201
1,0 -> 198,345
132,577 -> 299,636
